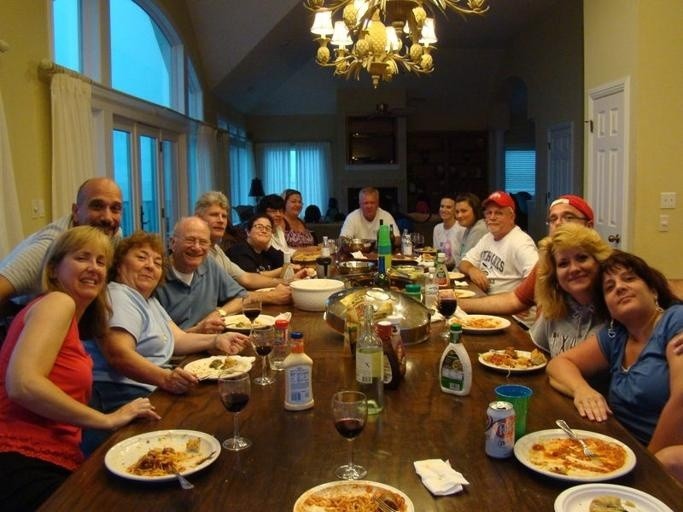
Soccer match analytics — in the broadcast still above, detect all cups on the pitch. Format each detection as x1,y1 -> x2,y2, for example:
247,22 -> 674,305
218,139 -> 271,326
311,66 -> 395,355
249,325 -> 275,385
331,391 -> 368,481
242,295 -> 262,326
413,236 -> 423,249
438,287 -> 457,338
217,370 -> 252,451
495,384 -> 533,438
411,233 -> 419,245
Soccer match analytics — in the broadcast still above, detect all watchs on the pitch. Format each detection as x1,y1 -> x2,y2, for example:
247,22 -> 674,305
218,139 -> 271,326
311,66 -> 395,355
215,306 -> 227,317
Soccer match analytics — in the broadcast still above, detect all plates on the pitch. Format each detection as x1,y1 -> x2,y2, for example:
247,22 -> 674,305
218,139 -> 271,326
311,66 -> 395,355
184,355 -> 252,381
428,272 -> 465,282
220,314 -> 276,332
103,429 -> 222,481
293,480 -> 414,512
478,349 -> 547,373
513,428 -> 636,482
553,483 -> 673,512
448,314 -> 511,335
439,288 -> 476,301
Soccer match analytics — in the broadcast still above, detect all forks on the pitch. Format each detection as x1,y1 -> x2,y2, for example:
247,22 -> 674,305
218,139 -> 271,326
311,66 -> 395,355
556,419 -> 596,458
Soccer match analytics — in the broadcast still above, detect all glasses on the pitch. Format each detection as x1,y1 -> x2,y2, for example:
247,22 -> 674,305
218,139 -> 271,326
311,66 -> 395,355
175,235 -> 211,247
548,213 -> 587,225
251,224 -> 272,232
484,209 -> 511,219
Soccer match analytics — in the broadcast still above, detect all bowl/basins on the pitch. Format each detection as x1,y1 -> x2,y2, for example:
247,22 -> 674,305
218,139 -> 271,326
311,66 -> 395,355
289,279 -> 344,312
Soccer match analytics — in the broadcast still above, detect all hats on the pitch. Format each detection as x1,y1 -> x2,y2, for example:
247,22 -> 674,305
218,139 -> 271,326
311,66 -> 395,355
482,190 -> 516,209
550,194 -> 595,224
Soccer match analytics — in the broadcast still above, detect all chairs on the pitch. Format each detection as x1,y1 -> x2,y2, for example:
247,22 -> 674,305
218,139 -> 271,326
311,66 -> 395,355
309,224 -> 340,243
231,205 -> 255,222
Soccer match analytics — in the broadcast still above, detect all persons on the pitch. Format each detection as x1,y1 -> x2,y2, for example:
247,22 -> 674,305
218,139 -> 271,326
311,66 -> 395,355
433,194 -> 463,262
195,192 -> 323,304
526,223 -> 613,360
0,177 -> 125,339
455,194 -> 594,326
282,189 -> 313,246
302,205 -> 321,225
256,197 -> 297,262
324,198 -> 339,225
73,232 -> 253,457
333,212 -> 347,224
459,191 -> 537,292
339,188 -> 402,249
1,225 -> 161,509
149,217 -> 250,331
455,192 -> 490,264
224,216 -> 303,279
545,255 -> 683,485
416,202 -> 430,214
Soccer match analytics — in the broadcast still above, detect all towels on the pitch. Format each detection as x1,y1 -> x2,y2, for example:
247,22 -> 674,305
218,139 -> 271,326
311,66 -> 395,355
414,459 -> 469,496
419,261 -> 434,267
277,310 -> 291,322
429,304 -> 467,322
454,280 -> 469,287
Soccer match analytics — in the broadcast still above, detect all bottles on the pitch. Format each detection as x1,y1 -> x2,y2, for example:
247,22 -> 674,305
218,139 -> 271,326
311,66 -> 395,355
434,249 -> 441,268
390,224 -> 396,250
269,320 -> 292,371
484,402 -> 515,458
279,251 -> 295,278
390,320 -> 406,376
405,284 -> 421,300
425,267 -> 440,309
356,305 -> 384,414
322,236 -> 331,257
377,219 -> 383,249
283,331 -> 314,411
432,253 -> 451,286
402,229 -> 407,252
404,235 -> 413,256
439,323 -> 473,396
378,225 -> 391,272
376,321 -> 399,389
374,256 -> 390,289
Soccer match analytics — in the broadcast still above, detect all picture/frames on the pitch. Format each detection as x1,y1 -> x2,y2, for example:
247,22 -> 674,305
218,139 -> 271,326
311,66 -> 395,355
346,112 -> 402,170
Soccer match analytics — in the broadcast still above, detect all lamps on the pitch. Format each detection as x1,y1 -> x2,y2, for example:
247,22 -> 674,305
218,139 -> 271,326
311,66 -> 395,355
301,0 -> 491,90
249,177 -> 265,207
